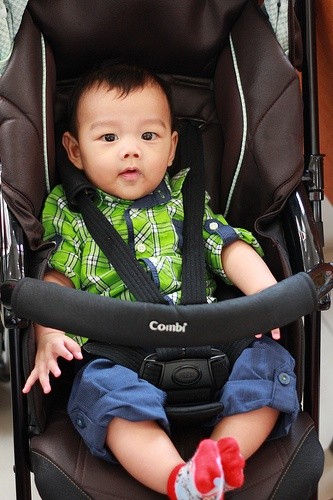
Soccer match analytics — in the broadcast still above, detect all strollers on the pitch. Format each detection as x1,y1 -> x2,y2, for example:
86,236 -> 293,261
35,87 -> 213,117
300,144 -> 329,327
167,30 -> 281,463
0,0 -> 333,500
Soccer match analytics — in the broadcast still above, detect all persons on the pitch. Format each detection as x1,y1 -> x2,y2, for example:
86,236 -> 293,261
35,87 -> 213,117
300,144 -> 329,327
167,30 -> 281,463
20,55 -> 301,500
